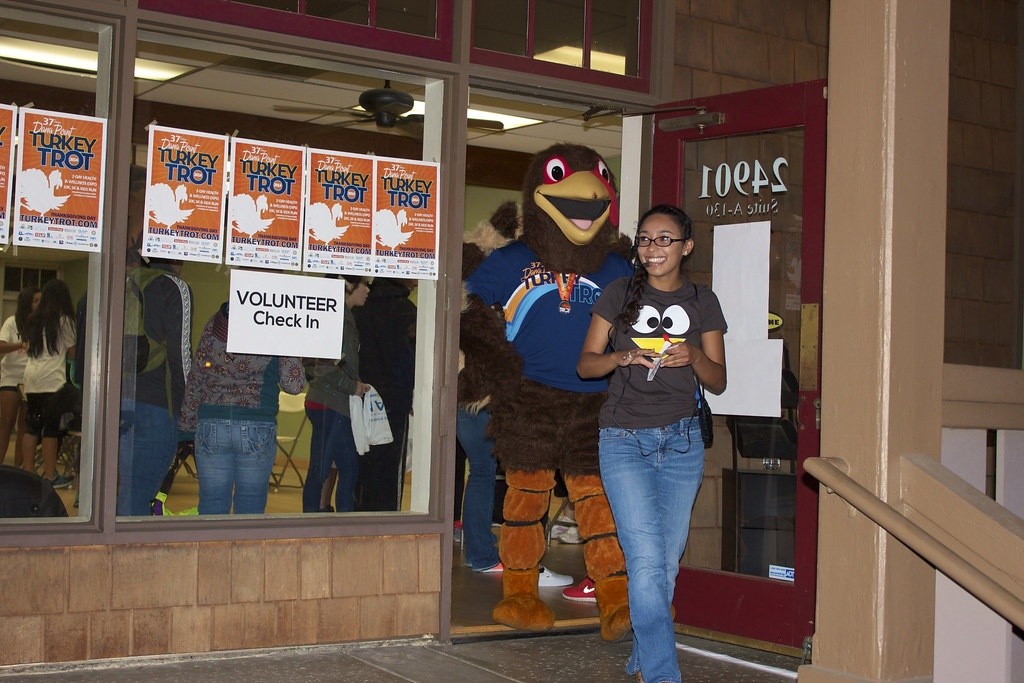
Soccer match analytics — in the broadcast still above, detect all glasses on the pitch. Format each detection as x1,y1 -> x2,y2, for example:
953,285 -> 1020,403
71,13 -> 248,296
635,236 -> 686,247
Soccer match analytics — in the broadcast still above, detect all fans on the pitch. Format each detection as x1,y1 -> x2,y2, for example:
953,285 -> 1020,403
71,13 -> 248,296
272,79 -> 503,134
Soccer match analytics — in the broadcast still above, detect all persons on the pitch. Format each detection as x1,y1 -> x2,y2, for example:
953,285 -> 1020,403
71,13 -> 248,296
116,231 -> 193,516
576,206 -> 728,683
535,470 -> 600,603
456,409 -> 504,572
0,287 -> 42,469
302,274 -> 417,514
176,302 -> 305,515
21,280 -> 76,487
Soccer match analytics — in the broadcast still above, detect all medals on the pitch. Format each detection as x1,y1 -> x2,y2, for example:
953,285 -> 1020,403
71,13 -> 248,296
558,301 -> 571,315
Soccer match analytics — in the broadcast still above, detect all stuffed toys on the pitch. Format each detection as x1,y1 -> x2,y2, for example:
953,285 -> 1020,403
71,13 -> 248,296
458,144 -> 676,643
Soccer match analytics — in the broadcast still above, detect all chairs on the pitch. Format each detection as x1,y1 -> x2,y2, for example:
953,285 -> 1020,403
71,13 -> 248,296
17,384 -> 80,476
269,391 -> 308,492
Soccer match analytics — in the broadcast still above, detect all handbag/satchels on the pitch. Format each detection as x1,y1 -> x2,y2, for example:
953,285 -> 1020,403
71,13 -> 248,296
363,384 -> 394,445
698,397 -> 714,449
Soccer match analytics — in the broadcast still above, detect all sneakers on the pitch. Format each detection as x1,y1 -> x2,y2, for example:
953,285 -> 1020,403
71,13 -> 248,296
538,566 -> 573,586
559,526 -> 585,543
44,474 -> 76,488
550,516 -> 576,538
562,577 -> 597,603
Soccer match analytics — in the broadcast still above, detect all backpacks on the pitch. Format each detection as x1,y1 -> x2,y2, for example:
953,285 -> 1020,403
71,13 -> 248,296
122,267 -> 167,380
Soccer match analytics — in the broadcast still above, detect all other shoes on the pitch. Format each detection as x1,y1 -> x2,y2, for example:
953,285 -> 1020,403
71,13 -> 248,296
624,659 -> 644,683
453,519 -> 462,542
483,562 -> 503,572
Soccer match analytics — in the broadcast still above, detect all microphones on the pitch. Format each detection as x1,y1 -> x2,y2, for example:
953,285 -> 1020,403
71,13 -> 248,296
627,246 -> 650,272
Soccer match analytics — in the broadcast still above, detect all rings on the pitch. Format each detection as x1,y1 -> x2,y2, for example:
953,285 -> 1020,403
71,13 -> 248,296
628,352 -> 631,358
